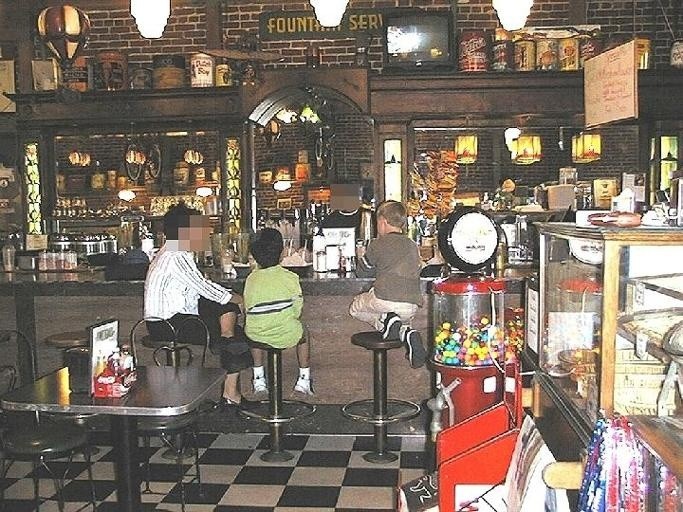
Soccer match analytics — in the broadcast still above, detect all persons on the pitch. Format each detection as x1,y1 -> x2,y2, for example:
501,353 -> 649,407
347,199 -> 427,370
241,227 -> 317,398
142,202 -> 263,412
315,182 -> 379,254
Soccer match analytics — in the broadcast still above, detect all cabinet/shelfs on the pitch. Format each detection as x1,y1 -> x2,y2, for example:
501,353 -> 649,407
528,220 -> 683,512
368,66 -> 682,266
2,88 -> 248,254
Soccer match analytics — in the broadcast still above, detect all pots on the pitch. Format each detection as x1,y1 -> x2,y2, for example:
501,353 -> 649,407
300,196 -> 330,237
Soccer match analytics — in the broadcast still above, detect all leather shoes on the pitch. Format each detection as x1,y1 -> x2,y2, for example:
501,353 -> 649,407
211,337 -> 248,356
220,395 -> 261,410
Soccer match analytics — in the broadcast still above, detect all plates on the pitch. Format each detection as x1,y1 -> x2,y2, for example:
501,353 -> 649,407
278,260 -> 312,269
234,262 -> 256,271
661,321 -> 682,358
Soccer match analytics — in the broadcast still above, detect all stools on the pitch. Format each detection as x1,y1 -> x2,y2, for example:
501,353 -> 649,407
339,331 -> 422,465
244,340 -> 317,462
141,332 -> 196,459
43,331 -> 100,458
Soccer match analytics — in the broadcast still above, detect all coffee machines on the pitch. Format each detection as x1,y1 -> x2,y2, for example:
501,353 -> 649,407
257,206 -> 298,247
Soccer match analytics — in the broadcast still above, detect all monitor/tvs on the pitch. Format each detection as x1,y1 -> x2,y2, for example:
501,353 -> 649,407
383,11 -> 454,72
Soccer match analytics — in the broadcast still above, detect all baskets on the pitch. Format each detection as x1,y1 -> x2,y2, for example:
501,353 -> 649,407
547,348 -> 599,398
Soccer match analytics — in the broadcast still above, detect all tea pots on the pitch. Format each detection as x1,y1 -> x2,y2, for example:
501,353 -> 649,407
203,195 -> 218,216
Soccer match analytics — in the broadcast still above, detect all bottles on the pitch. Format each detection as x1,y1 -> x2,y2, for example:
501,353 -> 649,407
215,161 -> 221,182
51,196 -> 89,219
96,343 -> 135,388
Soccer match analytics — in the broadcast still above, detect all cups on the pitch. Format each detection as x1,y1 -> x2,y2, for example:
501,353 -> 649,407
219,249 -> 235,276
231,233 -> 250,263
2,243 -> 17,271
65,343 -> 89,396
311,236 -> 367,271
211,171 -> 217,180
209,236 -> 230,264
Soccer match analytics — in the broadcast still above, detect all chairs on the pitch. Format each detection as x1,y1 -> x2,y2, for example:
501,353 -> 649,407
130,314 -> 211,512
0,331 -> 99,512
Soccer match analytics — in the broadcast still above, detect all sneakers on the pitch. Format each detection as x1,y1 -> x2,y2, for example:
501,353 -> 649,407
381,313 -> 401,341
251,376 -> 267,395
402,325 -> 427,369
293,378 -> 314,395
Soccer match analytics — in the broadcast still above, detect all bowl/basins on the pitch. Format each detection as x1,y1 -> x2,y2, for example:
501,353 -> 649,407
556,351 -> 598,374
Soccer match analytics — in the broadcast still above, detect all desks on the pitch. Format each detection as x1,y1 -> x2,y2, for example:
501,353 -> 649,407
0,361 -> 230,512
